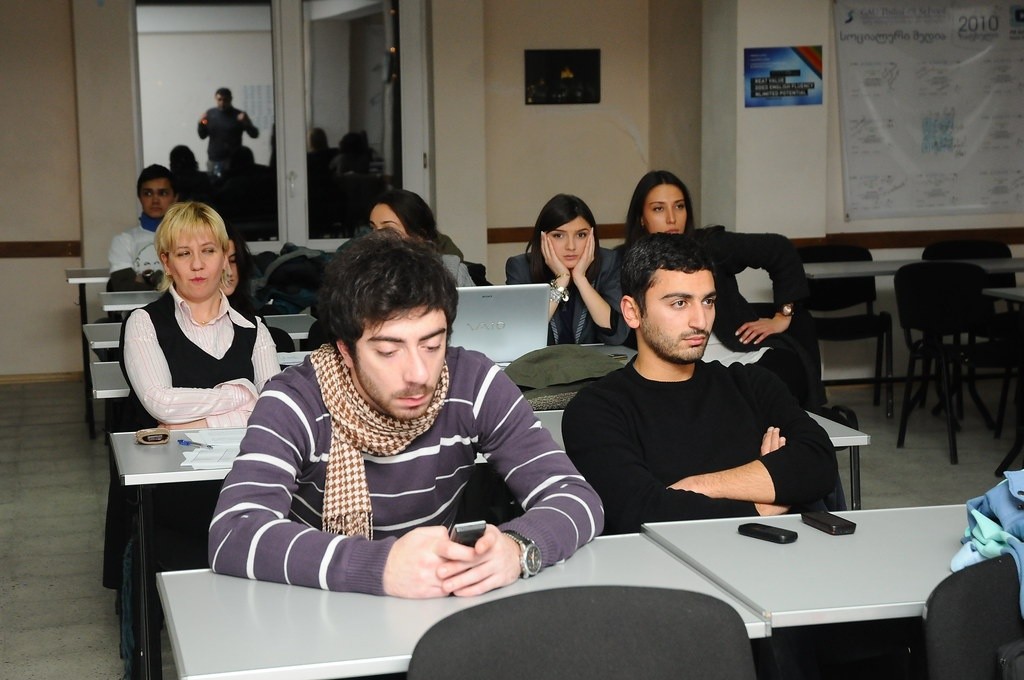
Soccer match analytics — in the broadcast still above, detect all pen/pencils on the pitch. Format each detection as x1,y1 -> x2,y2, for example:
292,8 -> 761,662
177,440 -> 214,450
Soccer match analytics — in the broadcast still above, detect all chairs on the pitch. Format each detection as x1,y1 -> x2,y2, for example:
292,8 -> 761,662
798,243 -> 896,419
406,586 -> 757,680
922,553 -> 1024,680
921,239 -> 1017,423
893,262 -> 998,465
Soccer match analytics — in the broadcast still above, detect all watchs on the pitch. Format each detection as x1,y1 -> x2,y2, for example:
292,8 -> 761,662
502,529 -> 542,580
782,303 -> 793,316
142,268 -> 156,286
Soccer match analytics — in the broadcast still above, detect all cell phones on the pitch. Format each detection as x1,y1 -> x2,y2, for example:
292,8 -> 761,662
802,511 -> 857,535
450,520 -> 488,548
738,523 -> 798,544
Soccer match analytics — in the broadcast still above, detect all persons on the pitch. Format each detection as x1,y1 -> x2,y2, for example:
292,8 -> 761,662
170,126 -> 385,238
506,194 -> 631,347
117,201 -> 282,429
613,171 -> 859,431
209,225 -> 605,597
219,232 -> 265,321
369,189 -> 476,287
112,164 -> 182,290
561,233 -> 838,532
198,90 -> 260,170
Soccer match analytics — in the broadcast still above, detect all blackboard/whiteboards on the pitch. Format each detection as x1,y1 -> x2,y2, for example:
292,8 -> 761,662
831,0 -> 1024,224
137,29 -> 275,173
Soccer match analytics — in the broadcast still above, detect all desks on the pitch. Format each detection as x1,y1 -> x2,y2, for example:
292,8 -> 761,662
67,256 -> 1024,680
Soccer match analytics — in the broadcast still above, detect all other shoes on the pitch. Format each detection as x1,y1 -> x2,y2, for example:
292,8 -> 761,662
134,618 -> 164,679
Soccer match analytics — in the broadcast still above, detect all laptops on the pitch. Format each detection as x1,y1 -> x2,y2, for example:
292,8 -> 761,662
448,283 -> 551,362
